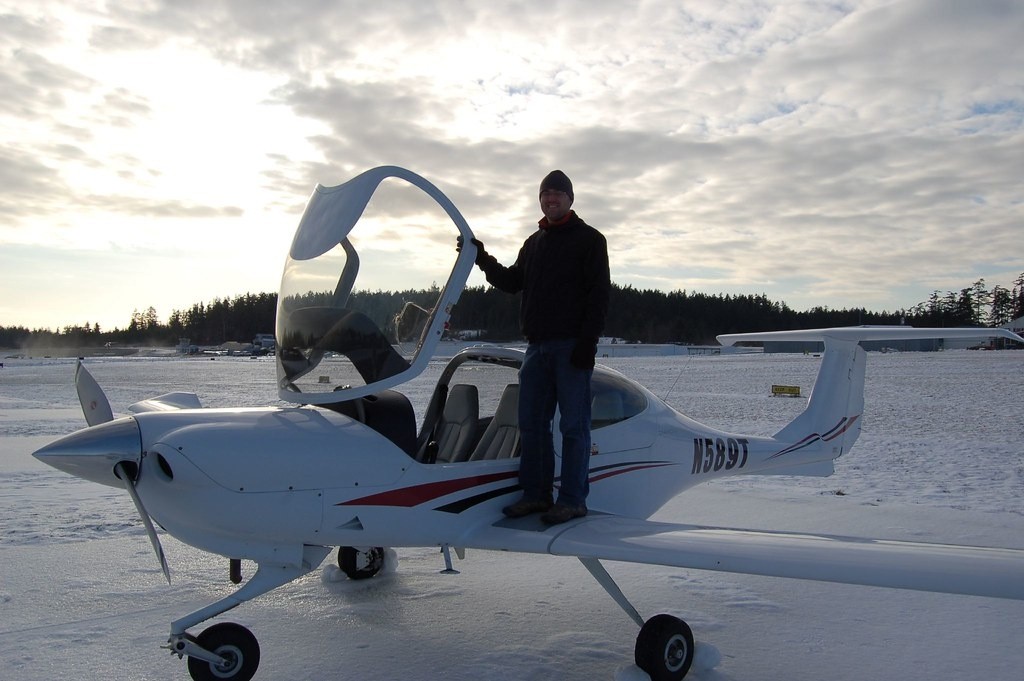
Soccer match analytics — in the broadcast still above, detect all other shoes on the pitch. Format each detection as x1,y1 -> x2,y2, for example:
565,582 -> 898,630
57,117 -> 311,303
541,498 -> 587,523
502,495 -> 553,518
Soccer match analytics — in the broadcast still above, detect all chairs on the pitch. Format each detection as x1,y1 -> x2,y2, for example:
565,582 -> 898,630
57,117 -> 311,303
428,384 -> 479,465
469,384 -> 522,461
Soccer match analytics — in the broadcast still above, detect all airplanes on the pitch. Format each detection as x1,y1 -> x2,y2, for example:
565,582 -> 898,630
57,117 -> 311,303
31,165 -> 1023,681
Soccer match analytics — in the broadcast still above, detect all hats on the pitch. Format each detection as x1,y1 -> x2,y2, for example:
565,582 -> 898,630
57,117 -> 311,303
539,170 -> 574,199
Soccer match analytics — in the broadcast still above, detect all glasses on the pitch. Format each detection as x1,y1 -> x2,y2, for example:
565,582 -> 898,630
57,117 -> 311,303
541,191 -> 571,200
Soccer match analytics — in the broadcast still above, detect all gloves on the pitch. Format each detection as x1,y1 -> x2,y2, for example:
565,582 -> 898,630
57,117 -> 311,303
573,338 -> 598,369
457,236 -> 487,265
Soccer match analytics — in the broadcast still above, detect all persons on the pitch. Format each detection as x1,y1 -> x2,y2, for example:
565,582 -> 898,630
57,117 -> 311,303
459,172 -> 610,528
